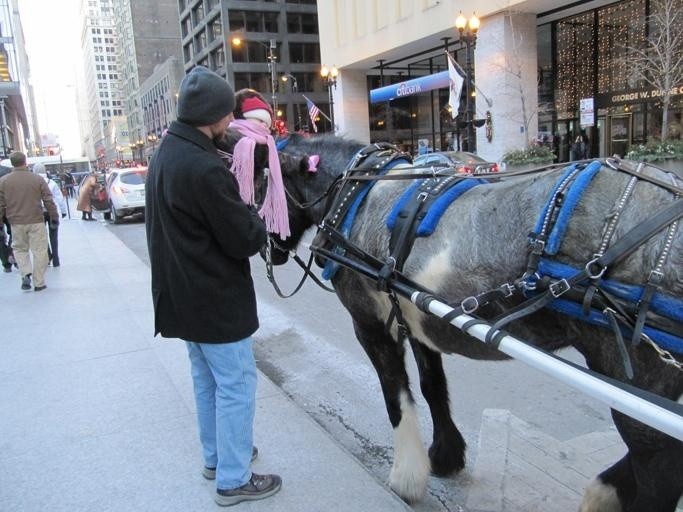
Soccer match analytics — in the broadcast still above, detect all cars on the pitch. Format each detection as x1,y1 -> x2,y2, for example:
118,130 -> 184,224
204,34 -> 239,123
71,166 -> 148,224
412,150 -> 499,174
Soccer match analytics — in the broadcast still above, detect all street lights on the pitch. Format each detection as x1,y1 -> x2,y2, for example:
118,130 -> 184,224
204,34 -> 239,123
282,74 -> 302,130
115,136 -> 157,161
319,65 -> 339,133
232,37 -> 279,121
455,10 -> 485,153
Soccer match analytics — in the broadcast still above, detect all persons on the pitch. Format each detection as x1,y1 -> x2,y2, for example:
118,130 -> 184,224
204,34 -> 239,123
159,88 -> 318,213
144,66 -> 283,507
0,147 -> 103,290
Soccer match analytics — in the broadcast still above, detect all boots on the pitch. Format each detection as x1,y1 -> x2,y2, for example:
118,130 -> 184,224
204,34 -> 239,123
82,213 -> 97,221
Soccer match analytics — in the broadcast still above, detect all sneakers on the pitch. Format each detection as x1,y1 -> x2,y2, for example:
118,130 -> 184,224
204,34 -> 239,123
202,445 -> 257,479
3,268 -> 11,273
21,283 -> 31,290
215,472 -> 281,506
34,285 -> 47,291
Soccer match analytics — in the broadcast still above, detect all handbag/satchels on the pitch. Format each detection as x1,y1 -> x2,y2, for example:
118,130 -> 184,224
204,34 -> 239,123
96,190 -> 107,202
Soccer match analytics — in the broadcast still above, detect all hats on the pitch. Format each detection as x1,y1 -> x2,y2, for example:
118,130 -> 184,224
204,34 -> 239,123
240,95 -> 272,127
1,159 -> 14,168
177,66 -> 236,124
33,164 -> 46,174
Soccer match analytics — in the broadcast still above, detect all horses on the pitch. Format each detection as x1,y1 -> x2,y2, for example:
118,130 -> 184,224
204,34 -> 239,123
249,130 -> 682,511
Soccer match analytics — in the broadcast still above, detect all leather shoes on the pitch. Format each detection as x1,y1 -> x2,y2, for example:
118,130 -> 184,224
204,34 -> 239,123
48,249 -> 60,267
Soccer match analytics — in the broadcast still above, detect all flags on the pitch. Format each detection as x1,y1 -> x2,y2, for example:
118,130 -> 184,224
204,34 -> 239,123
447,54 -> 464,119
306,98 -> 320,133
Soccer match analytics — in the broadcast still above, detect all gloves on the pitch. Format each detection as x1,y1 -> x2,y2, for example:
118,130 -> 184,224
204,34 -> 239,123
62,213 -> 67,219
305,154 -> 319,173
